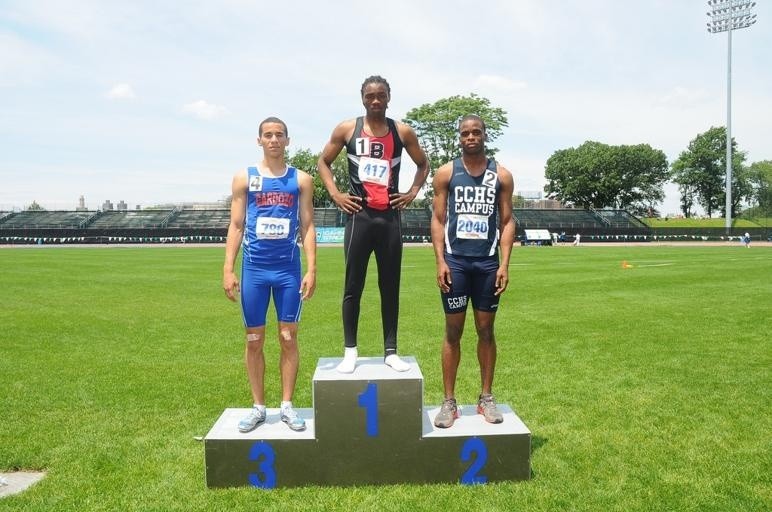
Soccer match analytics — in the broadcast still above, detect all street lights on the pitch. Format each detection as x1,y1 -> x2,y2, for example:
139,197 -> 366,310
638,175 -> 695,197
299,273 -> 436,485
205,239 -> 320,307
707,0 -> 757,236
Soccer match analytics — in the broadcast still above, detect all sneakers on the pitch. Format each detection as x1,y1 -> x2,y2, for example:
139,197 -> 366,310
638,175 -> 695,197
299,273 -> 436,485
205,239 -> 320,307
281,410 -> 306,431
239,407 -> 266,432
434,398 -> 457,428
477,396 -> 503,423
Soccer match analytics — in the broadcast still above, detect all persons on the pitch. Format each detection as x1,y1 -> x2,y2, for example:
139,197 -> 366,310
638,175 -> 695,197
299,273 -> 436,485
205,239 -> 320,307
223,117 -> 316,432
318,76 -> 430,373
552,232 -> 560,245
431,115 -> 516,428
744,232 -> 750,248
572,233 -> 580,247
560,230 -> 566,242
